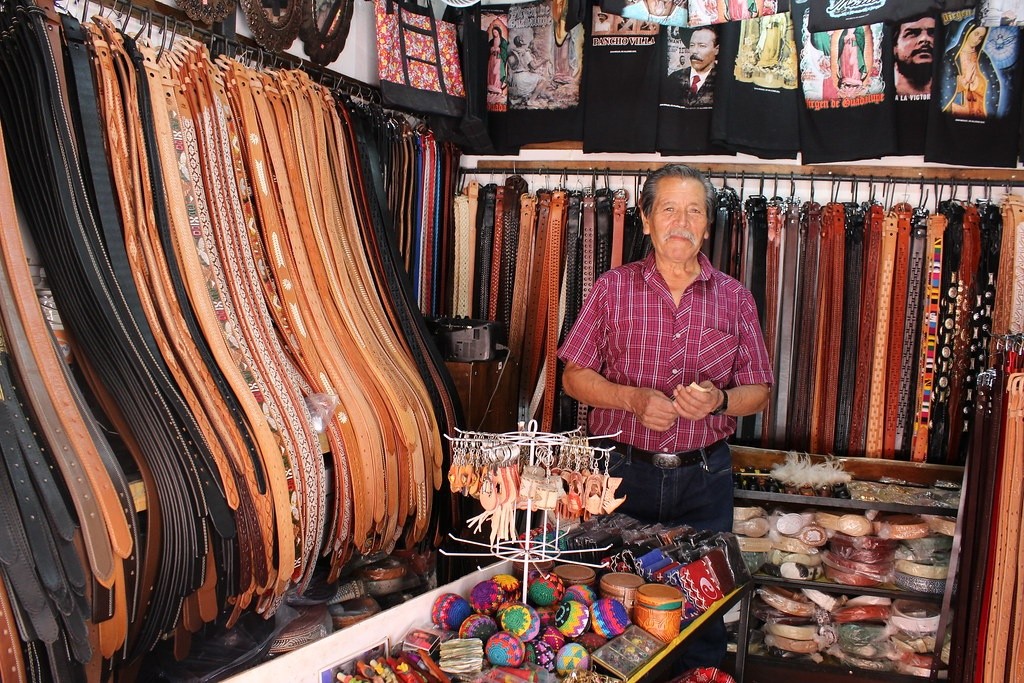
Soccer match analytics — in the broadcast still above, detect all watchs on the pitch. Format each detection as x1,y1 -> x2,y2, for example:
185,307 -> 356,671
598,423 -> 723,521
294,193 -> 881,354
709,390 -> 729,416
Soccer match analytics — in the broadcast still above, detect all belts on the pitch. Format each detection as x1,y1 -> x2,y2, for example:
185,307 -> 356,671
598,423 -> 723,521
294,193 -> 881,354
457,155 -> 1023,683
0,1 -> 470,683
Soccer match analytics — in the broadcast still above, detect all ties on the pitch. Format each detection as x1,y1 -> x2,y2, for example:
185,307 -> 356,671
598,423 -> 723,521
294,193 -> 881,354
691,76 -> 700,96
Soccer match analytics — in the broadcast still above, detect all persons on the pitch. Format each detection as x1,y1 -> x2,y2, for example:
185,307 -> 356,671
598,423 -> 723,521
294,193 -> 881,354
894,13 -> 934,101
555,165 -> 774,537
664,29 -> 720,109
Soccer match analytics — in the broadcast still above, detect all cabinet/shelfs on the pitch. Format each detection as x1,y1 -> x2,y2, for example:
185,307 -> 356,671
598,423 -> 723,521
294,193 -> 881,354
726,444 -> 968,683
445,357 -> 533,566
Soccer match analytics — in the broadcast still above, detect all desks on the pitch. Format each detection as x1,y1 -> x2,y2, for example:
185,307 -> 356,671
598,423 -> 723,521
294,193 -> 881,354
222,555 -> 754,683
